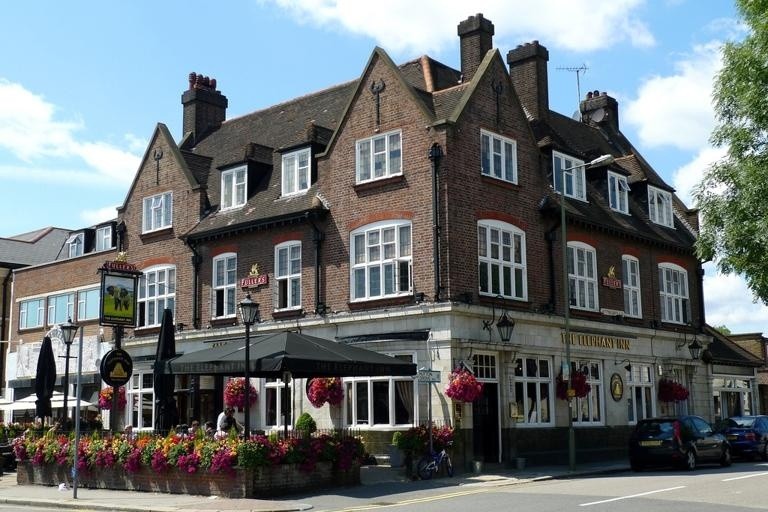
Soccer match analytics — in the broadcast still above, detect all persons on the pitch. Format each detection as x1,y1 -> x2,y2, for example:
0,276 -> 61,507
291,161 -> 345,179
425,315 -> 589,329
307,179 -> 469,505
219,409 -> 240,436
120,425 -> 138,441
215,406 -> 245,437
174,418 -> 216,439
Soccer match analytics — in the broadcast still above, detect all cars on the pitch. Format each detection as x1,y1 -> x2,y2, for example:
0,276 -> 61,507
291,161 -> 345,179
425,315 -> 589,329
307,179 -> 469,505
628,415 -> 767,471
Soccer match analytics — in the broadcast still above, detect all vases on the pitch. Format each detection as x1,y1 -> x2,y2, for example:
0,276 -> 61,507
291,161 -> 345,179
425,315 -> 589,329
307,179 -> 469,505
512,457 -> 528,470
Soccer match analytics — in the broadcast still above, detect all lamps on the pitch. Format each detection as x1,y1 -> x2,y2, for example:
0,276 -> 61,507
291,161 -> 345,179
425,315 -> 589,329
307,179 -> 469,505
615,359 -> 631,372
677,322 -> 704,360
482,295 -> 515,342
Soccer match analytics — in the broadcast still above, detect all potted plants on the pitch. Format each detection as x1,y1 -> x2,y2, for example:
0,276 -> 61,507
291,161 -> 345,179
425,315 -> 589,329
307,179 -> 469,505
471,455 -> 484,474
386,431 -> 407,468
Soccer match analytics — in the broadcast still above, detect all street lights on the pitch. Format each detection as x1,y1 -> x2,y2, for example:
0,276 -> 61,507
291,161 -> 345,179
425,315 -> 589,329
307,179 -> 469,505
58,315 -> 80,432
237,292 -> 260,439
560,153 -> 614,471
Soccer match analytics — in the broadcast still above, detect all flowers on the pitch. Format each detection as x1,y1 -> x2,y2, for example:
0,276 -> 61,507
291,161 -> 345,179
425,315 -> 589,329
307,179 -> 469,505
11,429 -> 365,480
224,377 -> 258,411
657,378 -> 690,403
307,377 -> 344,408
554,367 -> 591,402
446,368 -> 484,403
404,423 -> 457,448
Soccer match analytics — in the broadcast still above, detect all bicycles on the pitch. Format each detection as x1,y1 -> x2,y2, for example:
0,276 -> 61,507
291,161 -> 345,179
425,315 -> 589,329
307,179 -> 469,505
416,441 -> 455,479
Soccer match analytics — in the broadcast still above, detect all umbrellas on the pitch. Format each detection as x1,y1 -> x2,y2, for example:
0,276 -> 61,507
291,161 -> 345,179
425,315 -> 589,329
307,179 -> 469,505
151,305 -> 183,438
154,325 -> 419,438
33,334 -> 58,438
0,388 -> 93,415
